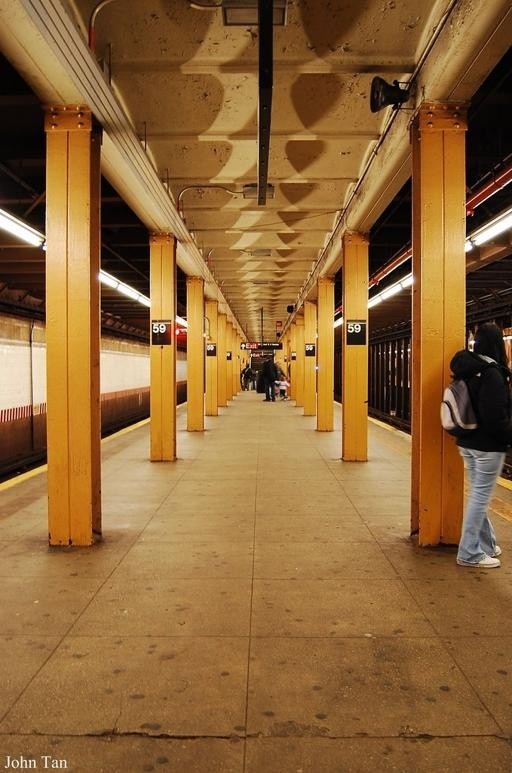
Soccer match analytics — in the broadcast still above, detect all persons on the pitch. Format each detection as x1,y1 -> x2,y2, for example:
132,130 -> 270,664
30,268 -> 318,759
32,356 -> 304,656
249,366 -> 258,389
260,353 -> 278,402
274,376 -> 290,400
275,362 -> 286,395
239,363 -> 251,390
447,318 -> 511,570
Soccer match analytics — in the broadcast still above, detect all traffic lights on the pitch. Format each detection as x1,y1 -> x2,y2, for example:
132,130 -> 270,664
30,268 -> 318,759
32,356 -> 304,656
175,328 -> 186,337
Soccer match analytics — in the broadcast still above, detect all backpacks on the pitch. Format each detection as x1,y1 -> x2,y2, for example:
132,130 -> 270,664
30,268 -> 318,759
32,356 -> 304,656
439,376 -> 479,437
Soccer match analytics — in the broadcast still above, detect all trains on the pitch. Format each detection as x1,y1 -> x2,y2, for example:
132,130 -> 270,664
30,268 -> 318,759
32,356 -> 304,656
1,308 -> 187,474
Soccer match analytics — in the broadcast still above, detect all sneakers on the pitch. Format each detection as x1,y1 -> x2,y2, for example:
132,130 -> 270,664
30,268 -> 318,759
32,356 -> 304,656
492,545 -> 501,557
263,398 -> 275,402
456,553 -> 501,569
